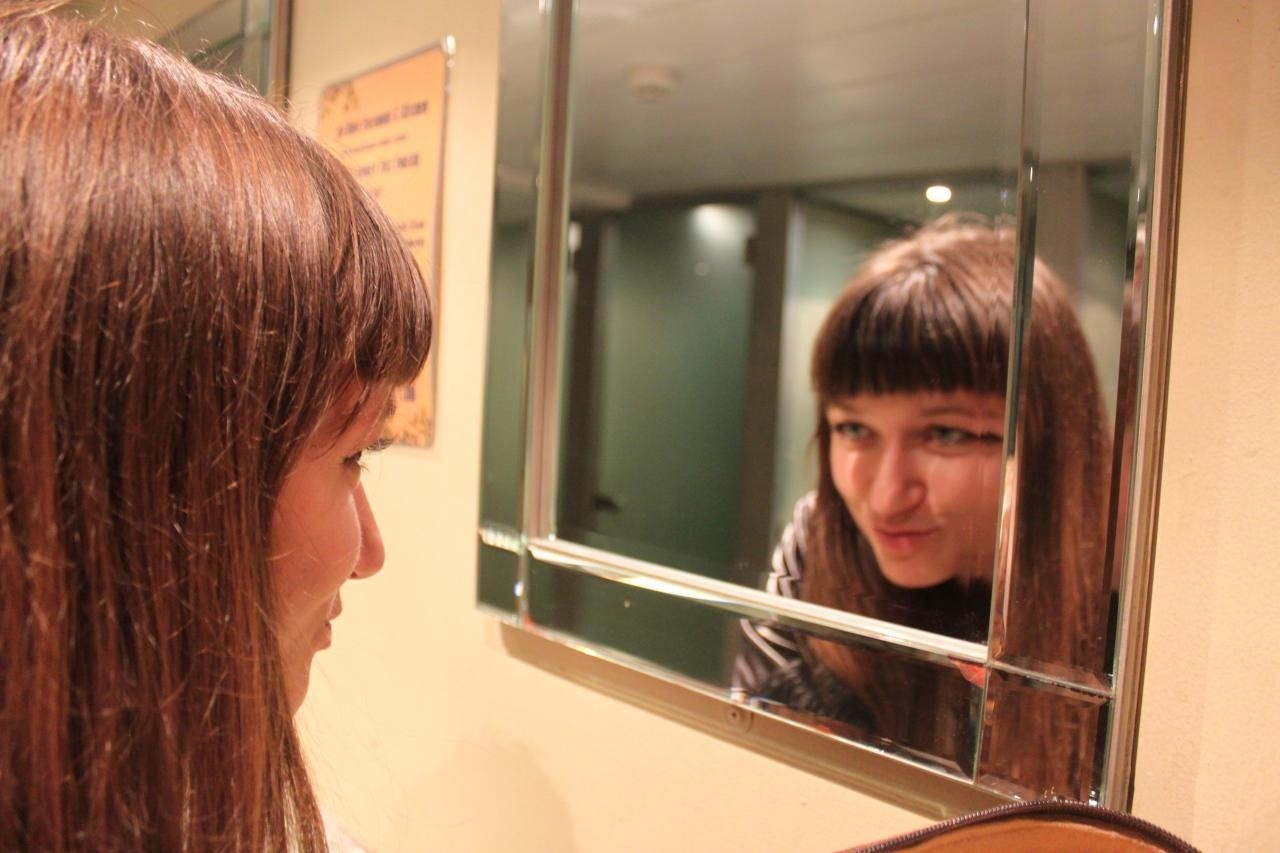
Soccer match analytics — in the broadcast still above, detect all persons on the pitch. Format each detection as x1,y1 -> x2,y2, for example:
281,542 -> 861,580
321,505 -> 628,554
0,8 -> 435,853
725,205 -> 1110,810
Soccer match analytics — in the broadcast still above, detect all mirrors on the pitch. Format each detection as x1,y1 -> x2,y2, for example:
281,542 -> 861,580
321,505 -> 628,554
472,0 -> 1197,829
